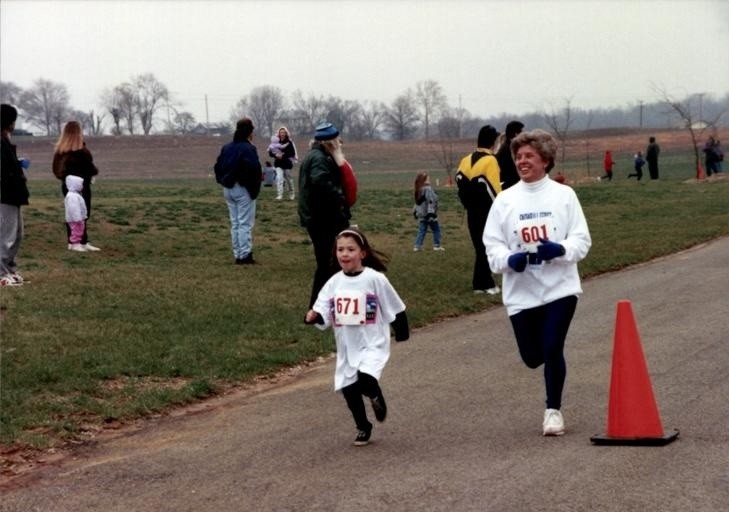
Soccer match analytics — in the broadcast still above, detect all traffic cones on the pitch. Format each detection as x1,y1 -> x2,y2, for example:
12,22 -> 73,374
696,160 -> 706,179
447,176 -> 452,186
588,301 -> 680,447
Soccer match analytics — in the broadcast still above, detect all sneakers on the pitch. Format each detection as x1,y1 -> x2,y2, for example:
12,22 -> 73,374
71,243 -> 87,252
82,242 -> 101,251
0,274 -> 22,286
353,430 -> 371,446
543,408 -> 565,437
11,271 -> 31,284
487,286 -> 500,295
371,395 -> 386,422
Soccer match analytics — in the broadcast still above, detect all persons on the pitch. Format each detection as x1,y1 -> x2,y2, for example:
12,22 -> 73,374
267,136 -> 290,160
262,160 -> 277,189
626,149 -> 646,181
268,125 -> 299,201
50,120 -> 104,253
301,225 -> 413,448
453,124 -> 507,296
297,118 -> 358,311
412,170 -> 450,253
479,131 -> 593,435
701,136 -> 725,176
494,132 -> 506,154
596,148 -> 617,182
496,118 -> 526,192
645,135 -> 661,180
62,171 -> 95,254
213,116 -> 265,265
0,100 -> 33,289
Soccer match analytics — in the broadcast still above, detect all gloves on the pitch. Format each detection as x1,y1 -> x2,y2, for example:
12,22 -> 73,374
537,238 -> 564,261
508,251 -> 529,272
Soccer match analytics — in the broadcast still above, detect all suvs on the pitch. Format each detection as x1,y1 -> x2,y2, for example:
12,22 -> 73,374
12,128 -> 33,136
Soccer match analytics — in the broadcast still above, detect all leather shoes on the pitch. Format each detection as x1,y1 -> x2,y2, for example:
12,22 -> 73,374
241,257 -> 262,265
235,258 -> 241,264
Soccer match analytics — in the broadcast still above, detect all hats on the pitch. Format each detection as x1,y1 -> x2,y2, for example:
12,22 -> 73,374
477,125 -> 501,147
315,122 -> 339,140
